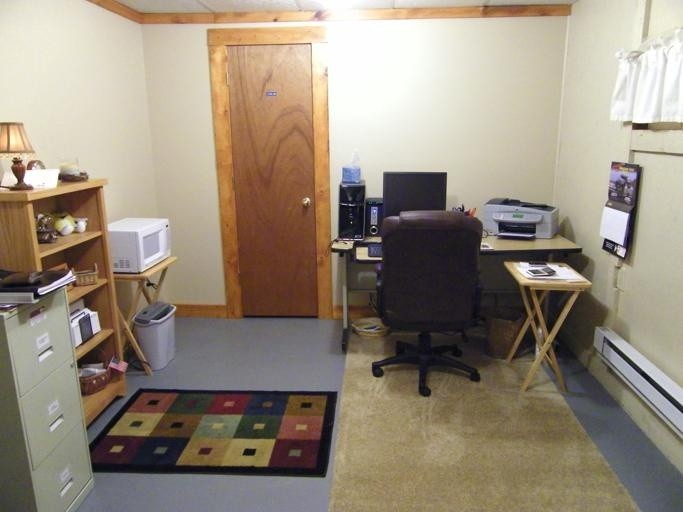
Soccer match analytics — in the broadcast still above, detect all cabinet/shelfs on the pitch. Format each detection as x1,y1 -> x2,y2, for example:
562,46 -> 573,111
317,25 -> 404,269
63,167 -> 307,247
0,177 -> 126,428
0,288 -> 96,512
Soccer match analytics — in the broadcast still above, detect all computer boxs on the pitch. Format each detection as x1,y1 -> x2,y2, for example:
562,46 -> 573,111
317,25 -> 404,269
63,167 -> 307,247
338,180 -> 366,240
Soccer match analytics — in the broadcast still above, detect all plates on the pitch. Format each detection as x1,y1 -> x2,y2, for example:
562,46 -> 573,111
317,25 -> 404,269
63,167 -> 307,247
353,316 -> 389,337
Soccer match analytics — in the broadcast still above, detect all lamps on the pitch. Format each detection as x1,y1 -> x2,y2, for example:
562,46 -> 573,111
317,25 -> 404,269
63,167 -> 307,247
0,120 -> 38,193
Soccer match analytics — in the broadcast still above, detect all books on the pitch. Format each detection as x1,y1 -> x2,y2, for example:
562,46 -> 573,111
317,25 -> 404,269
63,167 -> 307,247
0,269 -> 77,299
517,266 -> 580,280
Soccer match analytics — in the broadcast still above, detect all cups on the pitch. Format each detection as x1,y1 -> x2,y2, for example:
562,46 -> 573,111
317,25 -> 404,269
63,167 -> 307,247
486,307 -> 526,359
342,168 -> 361,183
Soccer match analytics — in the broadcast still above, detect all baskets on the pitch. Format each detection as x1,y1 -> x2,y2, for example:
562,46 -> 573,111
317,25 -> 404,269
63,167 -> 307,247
79,372 -> 108,395
72,263 -> 98,286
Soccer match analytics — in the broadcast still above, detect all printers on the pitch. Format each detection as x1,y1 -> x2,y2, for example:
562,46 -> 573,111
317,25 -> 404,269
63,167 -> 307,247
483,198 -> 560,239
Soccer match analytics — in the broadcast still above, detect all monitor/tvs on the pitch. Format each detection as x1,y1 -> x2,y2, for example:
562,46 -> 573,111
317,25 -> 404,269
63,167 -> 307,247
382,171 -> 448,217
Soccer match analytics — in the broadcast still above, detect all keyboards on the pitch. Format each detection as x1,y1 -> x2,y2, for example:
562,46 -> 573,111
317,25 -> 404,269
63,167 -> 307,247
367,243 -> 384,257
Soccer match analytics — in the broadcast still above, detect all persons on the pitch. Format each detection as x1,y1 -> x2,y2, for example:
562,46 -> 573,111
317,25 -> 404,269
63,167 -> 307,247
624,181 -> 633,205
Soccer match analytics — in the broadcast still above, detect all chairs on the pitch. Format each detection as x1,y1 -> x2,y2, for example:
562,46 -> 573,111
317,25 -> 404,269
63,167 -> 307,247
370,212 -> 481,394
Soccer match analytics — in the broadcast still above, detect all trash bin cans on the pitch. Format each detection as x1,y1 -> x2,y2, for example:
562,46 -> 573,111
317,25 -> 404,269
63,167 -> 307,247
131,302 -> 177,370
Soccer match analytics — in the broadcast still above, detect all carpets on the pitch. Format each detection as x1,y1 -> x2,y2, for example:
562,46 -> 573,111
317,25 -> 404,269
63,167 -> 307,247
326,301 -> 642,512
90,384 -> 337,478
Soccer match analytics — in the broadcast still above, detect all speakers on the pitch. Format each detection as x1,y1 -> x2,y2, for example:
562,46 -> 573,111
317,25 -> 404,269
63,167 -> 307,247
366,197 -> 383,237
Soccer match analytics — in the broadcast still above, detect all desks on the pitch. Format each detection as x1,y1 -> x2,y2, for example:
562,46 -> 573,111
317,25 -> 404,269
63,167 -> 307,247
504,259 -> 590,395
327,233 -> 584,351
109,256 -> 176,380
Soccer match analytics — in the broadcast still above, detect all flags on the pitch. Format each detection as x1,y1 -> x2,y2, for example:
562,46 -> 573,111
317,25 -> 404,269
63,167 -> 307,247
108,357 -> 128,372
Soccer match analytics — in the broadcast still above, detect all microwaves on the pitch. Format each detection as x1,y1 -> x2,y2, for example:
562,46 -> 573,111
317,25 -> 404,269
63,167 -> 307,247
106,217 -> 172,275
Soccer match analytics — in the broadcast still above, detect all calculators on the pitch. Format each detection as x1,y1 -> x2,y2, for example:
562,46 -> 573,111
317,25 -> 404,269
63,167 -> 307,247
527,267 -> 556,276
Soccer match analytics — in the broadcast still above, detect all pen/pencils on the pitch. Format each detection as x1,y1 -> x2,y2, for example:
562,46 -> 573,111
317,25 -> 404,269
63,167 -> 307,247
461,204 -> 477,217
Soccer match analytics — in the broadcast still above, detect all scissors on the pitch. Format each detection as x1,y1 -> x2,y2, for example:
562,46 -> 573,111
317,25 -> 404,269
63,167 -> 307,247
452,207 -> 461,212
